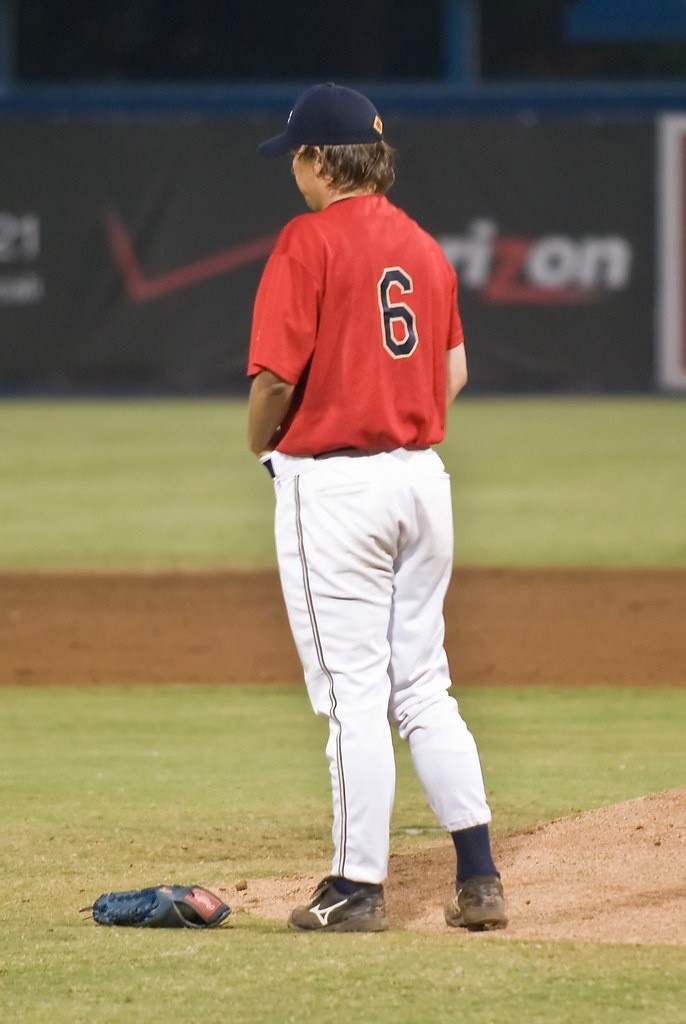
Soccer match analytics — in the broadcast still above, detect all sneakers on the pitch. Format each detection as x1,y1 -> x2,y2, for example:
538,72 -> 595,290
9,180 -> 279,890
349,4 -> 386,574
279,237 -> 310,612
443,876 -> 508,928
291,875 -> 388,932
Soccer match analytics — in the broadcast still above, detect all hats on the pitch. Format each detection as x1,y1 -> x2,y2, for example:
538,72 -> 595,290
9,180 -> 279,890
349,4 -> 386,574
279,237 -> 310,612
258,81 -> 383,162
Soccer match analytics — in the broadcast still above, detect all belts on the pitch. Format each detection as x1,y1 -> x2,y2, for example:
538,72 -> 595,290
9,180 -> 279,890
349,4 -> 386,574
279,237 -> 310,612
264,446 -> 356,478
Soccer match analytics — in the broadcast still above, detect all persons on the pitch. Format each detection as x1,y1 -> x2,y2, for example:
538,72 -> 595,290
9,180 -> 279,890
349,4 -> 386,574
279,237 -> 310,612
246,81 -> 509,932
